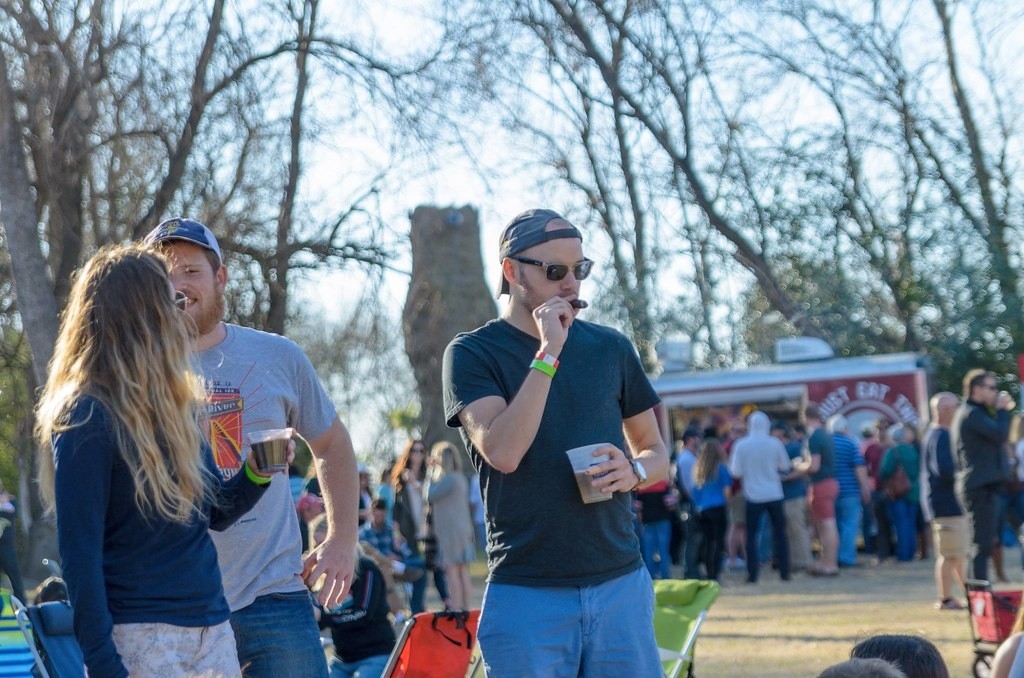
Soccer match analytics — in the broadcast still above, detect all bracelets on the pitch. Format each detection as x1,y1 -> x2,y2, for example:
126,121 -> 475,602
534,351 -> 560,369
241,456 -> 274,485
530,359 -> 560,376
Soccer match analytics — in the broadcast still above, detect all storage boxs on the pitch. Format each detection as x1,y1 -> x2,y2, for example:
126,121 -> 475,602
969,589 -> 1023,643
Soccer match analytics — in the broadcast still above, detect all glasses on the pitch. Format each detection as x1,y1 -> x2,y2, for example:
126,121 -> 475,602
510,255 -> 595,281
974,383 -> 1001,391
175,291 -> 188,312
410,447 -> 425,453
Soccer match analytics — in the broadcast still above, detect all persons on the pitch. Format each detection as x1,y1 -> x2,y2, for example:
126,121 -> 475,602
440,209 -> 671,677
388,439 -> 458,615
304,516 -> 397,678
948,369 -> 1016,591
142,216 -> 359,678
631,408 -> 926,584
0,488 -> 30,614
997,411 -> 1024,548
355,459 -> 423,624
424,442 -> 479,615
467,467 -> 490,562
922,390 -> 975,611
811,630 -> 1024,678
298,472 -> 325,533
36,243 -> 295,678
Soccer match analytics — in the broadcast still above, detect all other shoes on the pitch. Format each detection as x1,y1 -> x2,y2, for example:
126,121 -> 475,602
932,597 -> 962,609
807,568 -> 839,577
393,562 -> 424,582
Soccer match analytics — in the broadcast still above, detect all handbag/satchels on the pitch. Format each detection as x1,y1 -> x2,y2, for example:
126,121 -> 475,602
886,465 -> 912,500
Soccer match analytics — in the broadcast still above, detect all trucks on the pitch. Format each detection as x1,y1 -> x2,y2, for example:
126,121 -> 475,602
642,341 -> 931,453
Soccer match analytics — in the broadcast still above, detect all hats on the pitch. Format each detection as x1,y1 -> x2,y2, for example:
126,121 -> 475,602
357,462 -> 369,474
494,208 -> 583,300
144,217 -> 222,266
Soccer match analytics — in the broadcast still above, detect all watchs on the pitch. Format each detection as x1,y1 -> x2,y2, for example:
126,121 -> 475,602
628,457 -> 647,492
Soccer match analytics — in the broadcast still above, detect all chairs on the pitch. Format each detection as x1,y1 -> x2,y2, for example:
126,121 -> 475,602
650,578 -> 721,678
0,594 -> 90,678
380,609 -> 484,678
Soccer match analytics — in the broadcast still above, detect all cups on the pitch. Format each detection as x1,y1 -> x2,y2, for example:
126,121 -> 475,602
565,442 -> 613,504
246,428 -> 292,473
